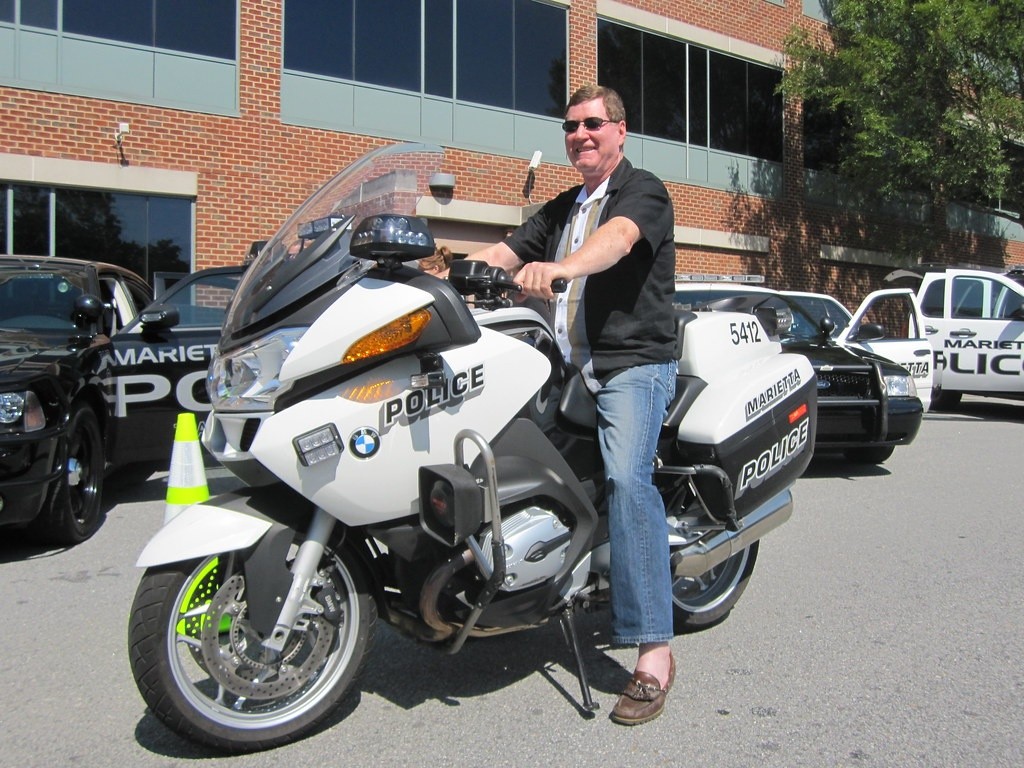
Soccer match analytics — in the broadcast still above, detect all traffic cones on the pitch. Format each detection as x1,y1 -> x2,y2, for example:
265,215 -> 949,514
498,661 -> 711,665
166,411 -> 236,637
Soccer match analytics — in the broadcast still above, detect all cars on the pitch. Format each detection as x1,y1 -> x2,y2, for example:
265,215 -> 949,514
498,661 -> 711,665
909,266 -> 1024,412
780,288 -> 934,414
669,271 -> 924,467
0,250 -> 262,544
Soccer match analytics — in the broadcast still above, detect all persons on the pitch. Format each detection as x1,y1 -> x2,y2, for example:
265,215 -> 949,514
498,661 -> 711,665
417,82 -> 681,725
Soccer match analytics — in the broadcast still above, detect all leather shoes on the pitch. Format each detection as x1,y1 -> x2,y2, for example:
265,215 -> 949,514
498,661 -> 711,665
612,649 -> 676,726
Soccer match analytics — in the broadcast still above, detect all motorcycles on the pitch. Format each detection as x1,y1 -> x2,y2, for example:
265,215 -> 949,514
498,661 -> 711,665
123,136 -> 822,752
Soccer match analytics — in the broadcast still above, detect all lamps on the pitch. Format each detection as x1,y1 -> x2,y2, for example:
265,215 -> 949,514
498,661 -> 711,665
428,173 -> 455,188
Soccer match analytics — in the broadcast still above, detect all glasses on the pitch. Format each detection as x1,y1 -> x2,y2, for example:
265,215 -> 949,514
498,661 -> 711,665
561,117 -> 621,132
